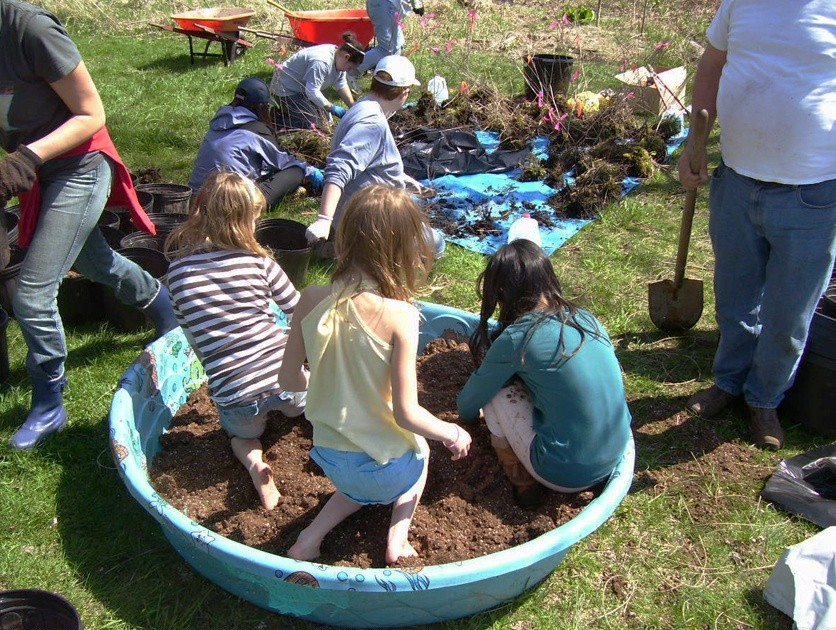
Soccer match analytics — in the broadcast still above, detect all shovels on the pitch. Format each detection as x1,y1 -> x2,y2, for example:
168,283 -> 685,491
648,109 -> 709,335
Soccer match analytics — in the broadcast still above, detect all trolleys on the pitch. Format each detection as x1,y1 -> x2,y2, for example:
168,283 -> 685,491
237,8 -> 375,47
149,8 -> 255,69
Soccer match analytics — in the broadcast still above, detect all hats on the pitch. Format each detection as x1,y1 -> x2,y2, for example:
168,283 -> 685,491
235,77 -> 276,105
374,55 -> 421,87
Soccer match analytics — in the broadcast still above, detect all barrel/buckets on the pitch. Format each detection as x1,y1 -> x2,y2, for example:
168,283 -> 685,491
0,588 -> 84,630
132,180 -> 192,215
523,54 -> 575,107
246,219 -> 316,295
119,230 -> 192,264
2,161 -> 155,380
128,214 -> 195,233
107,247 -> 173,335
821,263 -> 836,315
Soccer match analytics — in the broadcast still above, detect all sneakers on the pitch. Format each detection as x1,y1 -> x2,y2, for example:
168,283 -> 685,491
686,384 -> 736,416
749,407 -> 785,450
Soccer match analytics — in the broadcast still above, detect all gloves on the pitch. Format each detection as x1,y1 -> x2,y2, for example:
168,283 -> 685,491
305,214 -> 334,247
404,174 -> 424,194
411,0 -> 425,17
0,144 -> 41,208
327,104 -> 346,118
310,167 -> 325,189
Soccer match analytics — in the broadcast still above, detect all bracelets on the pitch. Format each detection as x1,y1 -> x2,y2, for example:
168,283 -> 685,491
442,424 -> 459,448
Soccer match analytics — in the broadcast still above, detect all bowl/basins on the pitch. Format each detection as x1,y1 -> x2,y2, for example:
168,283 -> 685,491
111,289 -> 636,629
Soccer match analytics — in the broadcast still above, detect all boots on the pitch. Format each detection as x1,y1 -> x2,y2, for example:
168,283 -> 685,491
135,278 -> 179,349
10,366 -> 67,450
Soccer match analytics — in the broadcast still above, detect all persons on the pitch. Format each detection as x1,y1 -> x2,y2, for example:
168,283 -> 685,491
346,0 -> 424,94
0,0 -> 179,452
304,55 -> 445,261
678,0 -> 836,448
456,239 -> 632,492
167,172 -> 310,510
276,183 -> 472,562
189,77 -> 324,212
270,32 -> 365,130
